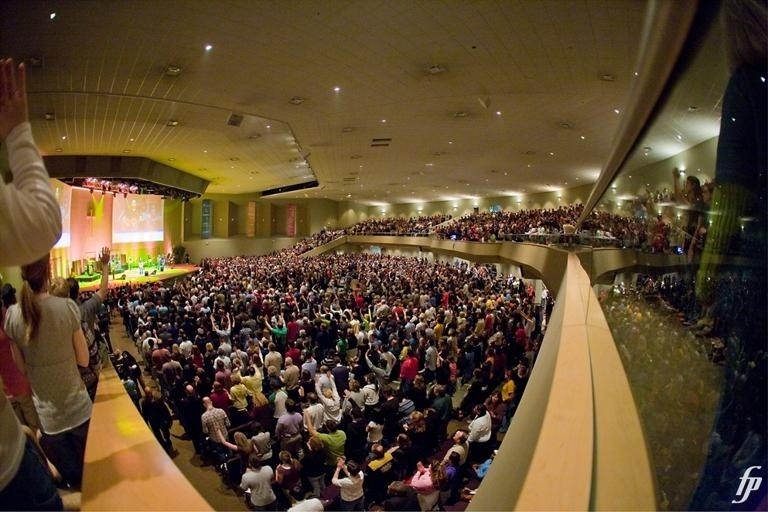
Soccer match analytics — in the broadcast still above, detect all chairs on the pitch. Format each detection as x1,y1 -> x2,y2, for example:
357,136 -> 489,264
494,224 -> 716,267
221,419 -> 484,511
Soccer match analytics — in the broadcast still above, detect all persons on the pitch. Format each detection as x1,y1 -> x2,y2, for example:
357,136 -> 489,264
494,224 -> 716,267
49,246 -> 111,402
2,281 -> 17,306
3,252 -> 90,488
67,277 -> 81,302
0,57 -> 64,511
77,0 -> 768,510
0,300 -> 39,432
